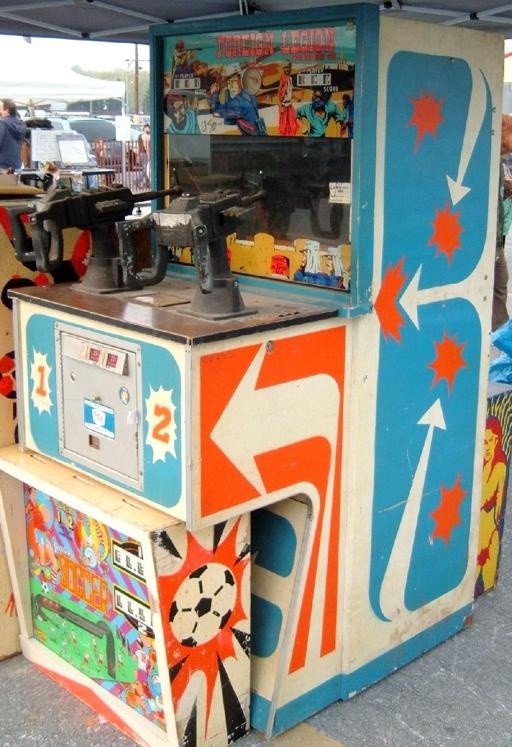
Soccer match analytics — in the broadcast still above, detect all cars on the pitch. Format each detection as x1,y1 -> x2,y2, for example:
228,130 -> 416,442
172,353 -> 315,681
14,104 -> 149,190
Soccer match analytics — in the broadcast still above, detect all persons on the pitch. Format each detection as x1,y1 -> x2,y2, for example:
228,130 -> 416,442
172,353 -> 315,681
474,417 -> 506,597
0,97 -> 26,176
132,124 -> 151,188
